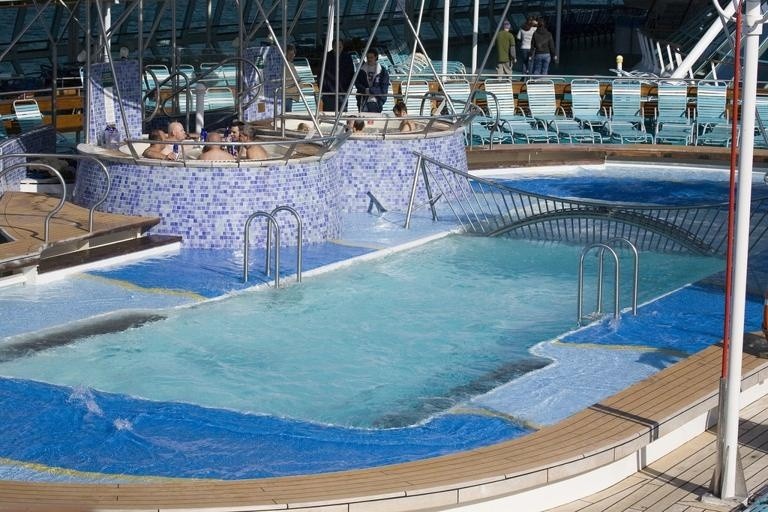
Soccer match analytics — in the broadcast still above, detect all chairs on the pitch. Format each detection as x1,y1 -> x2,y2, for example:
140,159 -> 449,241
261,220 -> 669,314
0,52 -> 741,149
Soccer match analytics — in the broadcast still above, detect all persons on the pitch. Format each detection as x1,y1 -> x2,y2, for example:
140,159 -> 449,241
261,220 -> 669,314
285,44 -> 302,113
495,20 -> 517,82
346,115 -> 358,133
352,118 -> 365,133
142,121 -> 269,161
317,38 -> 354,113
517,16 -> 538,82
393,102 -> 416,133
297,123 -> 311,131
429,107 -> 449,131
355,49 -> 389,112
529,20 -> 557,75
97,124 -> 133,158
